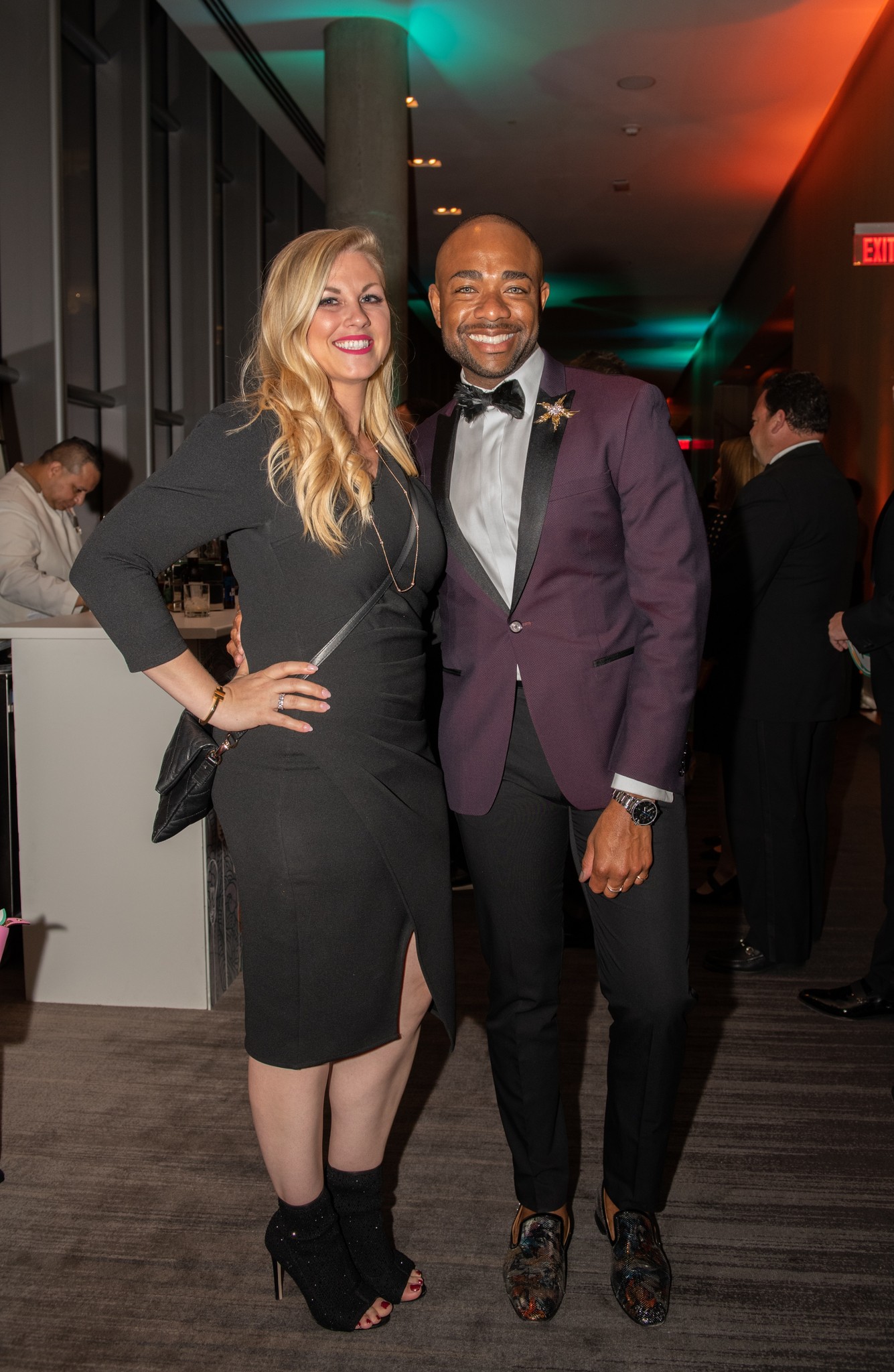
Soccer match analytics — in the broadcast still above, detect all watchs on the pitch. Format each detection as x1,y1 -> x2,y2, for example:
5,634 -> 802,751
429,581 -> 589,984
611,787 -> 659,826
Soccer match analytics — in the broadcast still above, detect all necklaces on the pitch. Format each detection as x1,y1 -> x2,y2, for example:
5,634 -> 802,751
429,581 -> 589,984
362,423 -> 421,592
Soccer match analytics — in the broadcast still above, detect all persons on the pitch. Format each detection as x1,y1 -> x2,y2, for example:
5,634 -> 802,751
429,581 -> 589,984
0,438 -> 112,621
698,368 -> 862,982
703,476 -> 721,541
229,212 -> 709,1329
65,221 -> 447,1329
841,474 -> 868,717
799,486 -> 894,1020
695,437 -> 772,909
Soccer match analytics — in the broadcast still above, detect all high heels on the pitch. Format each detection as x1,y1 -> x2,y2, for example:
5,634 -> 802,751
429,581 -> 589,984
322,1157 -> 427,1302
265,1189 -> 392,1332
690,871 -> 741,902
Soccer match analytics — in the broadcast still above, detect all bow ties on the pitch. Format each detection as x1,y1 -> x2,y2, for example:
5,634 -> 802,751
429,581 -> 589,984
454,378 -> 526,422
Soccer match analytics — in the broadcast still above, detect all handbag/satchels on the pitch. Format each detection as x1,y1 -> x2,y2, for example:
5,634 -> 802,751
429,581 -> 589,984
152,668 -> 237,842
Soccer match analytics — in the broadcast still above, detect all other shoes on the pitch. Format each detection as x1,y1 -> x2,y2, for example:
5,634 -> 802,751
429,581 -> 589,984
702,835 -> 721,845
699,846 -> 721,859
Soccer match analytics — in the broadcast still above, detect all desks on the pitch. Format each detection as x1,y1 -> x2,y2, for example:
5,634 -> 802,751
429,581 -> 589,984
1,604 -> 241,1010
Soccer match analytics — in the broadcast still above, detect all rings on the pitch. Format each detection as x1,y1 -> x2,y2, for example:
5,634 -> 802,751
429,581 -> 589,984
606,883 -> 623,893
278,693 -> 285,710
637,874 -> 648,881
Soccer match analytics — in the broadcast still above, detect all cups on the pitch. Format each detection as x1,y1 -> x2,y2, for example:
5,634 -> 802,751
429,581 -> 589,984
183,583 -> 211,617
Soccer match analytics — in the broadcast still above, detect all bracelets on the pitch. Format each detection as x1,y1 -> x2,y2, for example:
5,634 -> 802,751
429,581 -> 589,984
198,686 -> 225,726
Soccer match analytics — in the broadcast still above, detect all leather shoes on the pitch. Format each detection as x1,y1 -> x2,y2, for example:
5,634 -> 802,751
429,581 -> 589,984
501,1201 -> 571,1321
595,1185 -> 671,1326
796,976 -> 894,1022
703,940 -> 804,975
